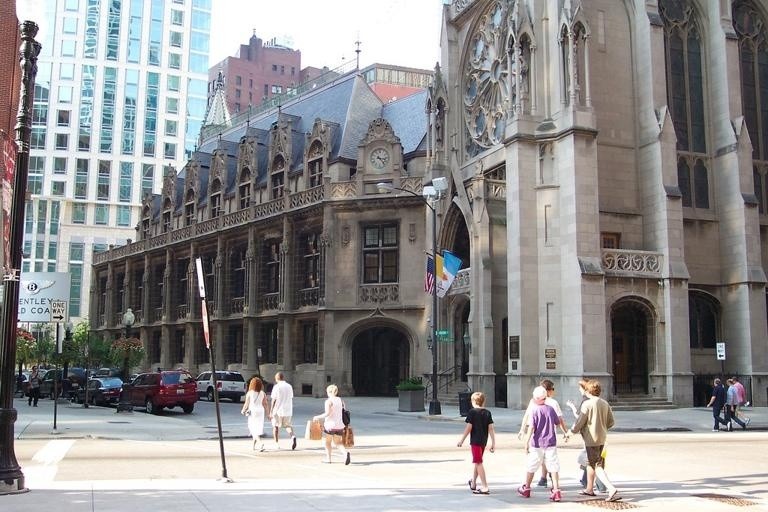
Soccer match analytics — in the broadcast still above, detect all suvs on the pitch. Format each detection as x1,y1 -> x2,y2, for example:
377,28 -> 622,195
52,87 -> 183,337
11,365 -> 249,418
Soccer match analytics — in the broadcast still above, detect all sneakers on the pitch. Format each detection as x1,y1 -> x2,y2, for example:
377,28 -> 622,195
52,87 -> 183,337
513,484 -> 532,498
536,477 -> 548,487
711,417 -> 750,432
549,489 -> 562,502
321,451 -> 352,465
252,436 -> 298,452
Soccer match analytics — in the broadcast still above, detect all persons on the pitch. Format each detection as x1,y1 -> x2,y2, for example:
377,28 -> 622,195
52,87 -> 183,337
518,379 -> 568,488
707,376 -> 750,431
565,379 -> 623,503
457,392 -> 495,493
516,386 -> 568,501
567,379 -> 606,493
29,366 -> 41,406
314,384 -> 350,465
270,373 -> 296,450
241,377 -> 270,452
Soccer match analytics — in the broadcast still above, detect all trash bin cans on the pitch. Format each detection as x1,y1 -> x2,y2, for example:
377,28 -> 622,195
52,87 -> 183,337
458,391 -> 474,417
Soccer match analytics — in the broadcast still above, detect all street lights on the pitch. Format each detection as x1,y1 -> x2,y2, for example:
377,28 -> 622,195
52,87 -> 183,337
114,304 -> 136,413
375,175 -> 449,417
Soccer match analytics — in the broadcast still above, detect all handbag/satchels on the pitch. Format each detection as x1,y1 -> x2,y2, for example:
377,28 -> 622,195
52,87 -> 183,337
341,409 -> 352,427
343,427 -> 355,449
304,419 -> 323,441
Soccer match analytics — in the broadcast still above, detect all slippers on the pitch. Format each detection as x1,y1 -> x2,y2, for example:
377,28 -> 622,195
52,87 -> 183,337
578,489 -> 597,496
469,479 -> 477,491
471,488 -> 490,495
605,496 -> 622,502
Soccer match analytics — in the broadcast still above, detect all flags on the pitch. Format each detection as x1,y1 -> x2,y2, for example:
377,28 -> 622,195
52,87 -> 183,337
436,254 -> 443,293
425,257 -> 434,295
436,250 -> 463,298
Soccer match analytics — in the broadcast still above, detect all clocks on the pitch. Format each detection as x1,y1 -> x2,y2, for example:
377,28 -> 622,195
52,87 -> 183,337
370,148 -> 389,169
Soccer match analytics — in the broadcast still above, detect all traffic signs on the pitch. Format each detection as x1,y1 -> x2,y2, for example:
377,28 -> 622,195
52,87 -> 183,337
714,342 -> 726,362
49,299 -> 68,325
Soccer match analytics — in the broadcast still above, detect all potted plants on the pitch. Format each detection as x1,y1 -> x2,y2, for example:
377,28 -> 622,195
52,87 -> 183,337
395,377 -> 425,412
